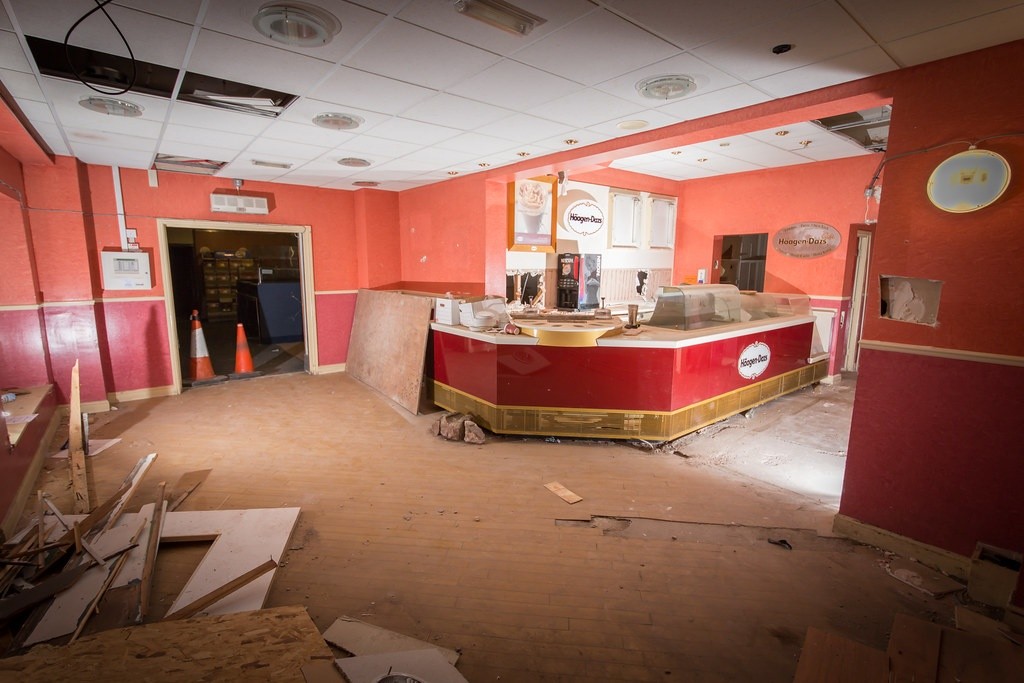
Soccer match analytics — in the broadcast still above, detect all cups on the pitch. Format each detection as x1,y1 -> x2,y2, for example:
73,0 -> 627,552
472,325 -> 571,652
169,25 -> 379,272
627,304 -> 638,326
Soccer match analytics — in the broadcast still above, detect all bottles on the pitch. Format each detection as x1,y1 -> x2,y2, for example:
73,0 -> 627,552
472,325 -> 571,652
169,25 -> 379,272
503,322 -> 520,335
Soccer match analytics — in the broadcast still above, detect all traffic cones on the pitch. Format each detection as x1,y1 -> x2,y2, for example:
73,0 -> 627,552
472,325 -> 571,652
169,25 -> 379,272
181,309 -> 228,388
233,322 -> 254,375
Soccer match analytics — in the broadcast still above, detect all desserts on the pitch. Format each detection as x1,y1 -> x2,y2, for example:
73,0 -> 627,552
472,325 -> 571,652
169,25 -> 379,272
519,183 -> 546,213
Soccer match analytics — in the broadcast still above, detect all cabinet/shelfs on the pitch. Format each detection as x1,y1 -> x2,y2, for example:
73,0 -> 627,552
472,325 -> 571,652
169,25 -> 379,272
202,251 -> 259,322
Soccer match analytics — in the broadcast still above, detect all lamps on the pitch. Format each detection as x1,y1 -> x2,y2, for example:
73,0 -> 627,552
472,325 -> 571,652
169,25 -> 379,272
312,113 -> 359,130
77,96 -> 143,117
253,0 -> 344,49
635,74 -> 697,102
